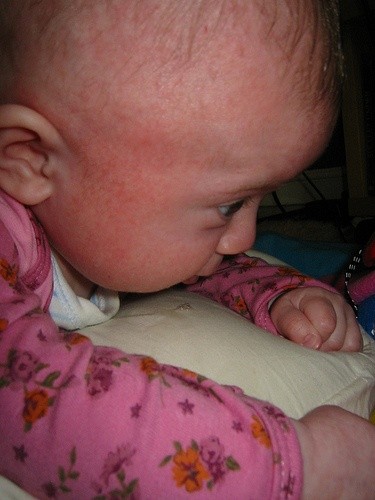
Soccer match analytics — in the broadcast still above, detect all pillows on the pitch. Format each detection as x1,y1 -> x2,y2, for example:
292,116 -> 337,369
1,245 -> 375,499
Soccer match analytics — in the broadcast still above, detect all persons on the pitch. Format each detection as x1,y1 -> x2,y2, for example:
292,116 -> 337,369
0,1 -> 373,500
